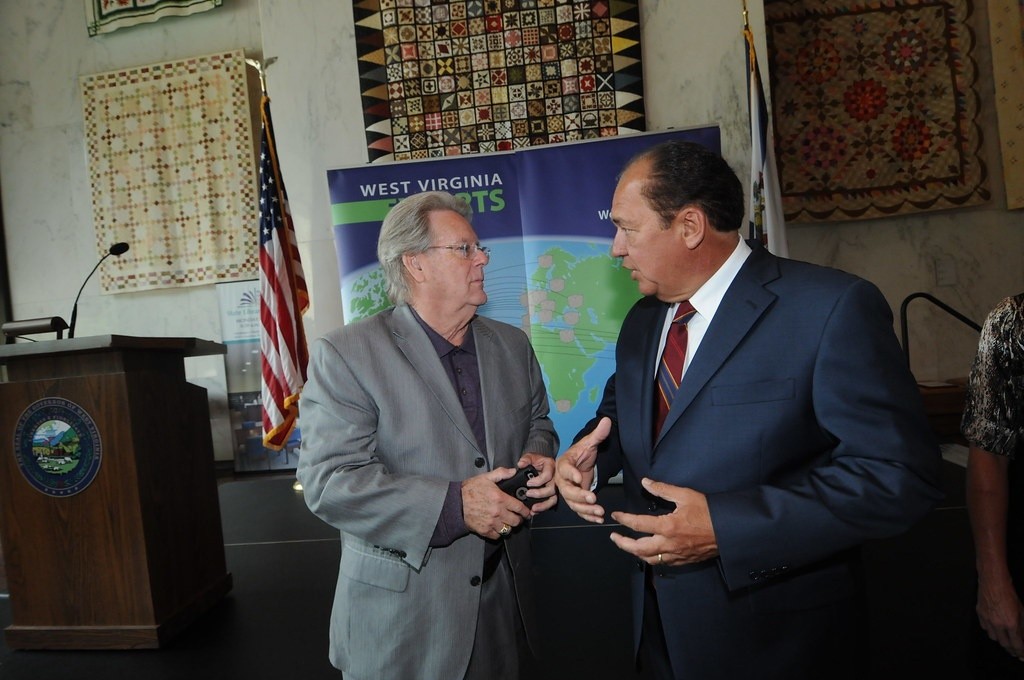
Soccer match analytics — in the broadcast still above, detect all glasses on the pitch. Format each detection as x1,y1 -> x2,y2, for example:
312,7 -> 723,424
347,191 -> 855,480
420,241 -> 491,262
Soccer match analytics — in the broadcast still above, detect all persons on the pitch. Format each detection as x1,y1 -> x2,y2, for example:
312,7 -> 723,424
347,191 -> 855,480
555,140 -> 943,680
297,191 -> 561,680
964,294 -> 1024,663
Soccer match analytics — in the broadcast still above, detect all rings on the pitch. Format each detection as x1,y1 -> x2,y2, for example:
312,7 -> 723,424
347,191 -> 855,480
500,524 -> 511,536
658,553 -> 665,567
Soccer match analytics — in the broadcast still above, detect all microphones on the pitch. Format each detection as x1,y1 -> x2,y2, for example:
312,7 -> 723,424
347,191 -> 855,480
68,243 -> 130,339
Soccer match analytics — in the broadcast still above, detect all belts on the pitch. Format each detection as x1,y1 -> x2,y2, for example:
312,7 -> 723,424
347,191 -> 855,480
482,545 -> 504,582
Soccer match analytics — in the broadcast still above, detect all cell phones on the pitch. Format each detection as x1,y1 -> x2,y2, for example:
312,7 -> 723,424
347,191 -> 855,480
496,464 -> 549,509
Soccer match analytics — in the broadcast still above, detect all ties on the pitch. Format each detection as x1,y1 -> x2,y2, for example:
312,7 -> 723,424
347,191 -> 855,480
652,300 -> 697,443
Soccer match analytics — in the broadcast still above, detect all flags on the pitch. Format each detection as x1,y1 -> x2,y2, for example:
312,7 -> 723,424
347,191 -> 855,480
743,25 -> 789,261
258,91 -> 310,453
